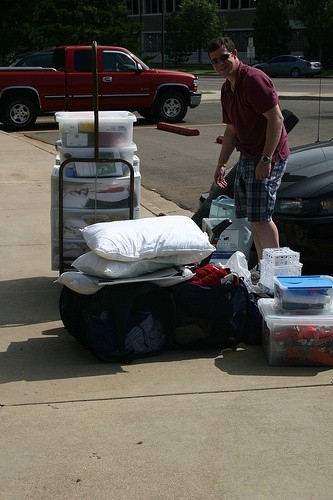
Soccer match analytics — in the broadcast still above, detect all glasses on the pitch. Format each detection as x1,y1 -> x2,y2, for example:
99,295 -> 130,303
210,52 -> 232,64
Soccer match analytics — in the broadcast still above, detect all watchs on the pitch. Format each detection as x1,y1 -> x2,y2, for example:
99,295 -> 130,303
261,156 -> 272,163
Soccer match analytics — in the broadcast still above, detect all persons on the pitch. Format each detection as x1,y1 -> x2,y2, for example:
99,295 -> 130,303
207,37 -> 290,295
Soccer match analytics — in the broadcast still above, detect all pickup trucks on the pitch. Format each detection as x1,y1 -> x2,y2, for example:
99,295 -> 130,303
0,45 -> 202,132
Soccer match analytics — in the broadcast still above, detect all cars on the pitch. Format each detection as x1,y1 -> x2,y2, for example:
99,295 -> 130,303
251,55 -> 323,78
198,136 -> 333,277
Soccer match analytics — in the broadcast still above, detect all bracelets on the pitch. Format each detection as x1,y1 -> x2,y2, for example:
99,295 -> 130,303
217,163 -> 227,167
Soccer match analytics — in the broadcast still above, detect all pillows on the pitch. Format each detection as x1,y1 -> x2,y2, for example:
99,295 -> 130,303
79,215 -> 217,265
71,232 -> 215,279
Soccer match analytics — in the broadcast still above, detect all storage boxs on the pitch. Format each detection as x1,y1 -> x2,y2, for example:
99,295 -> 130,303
48,110 -> 141,272
203,193 -> 333,369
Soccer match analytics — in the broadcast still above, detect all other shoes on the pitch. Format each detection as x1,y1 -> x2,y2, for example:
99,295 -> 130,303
250,263 -> 260,280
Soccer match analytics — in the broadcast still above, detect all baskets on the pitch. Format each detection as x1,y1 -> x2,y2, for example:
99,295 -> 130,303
259,246 -> 303,289
